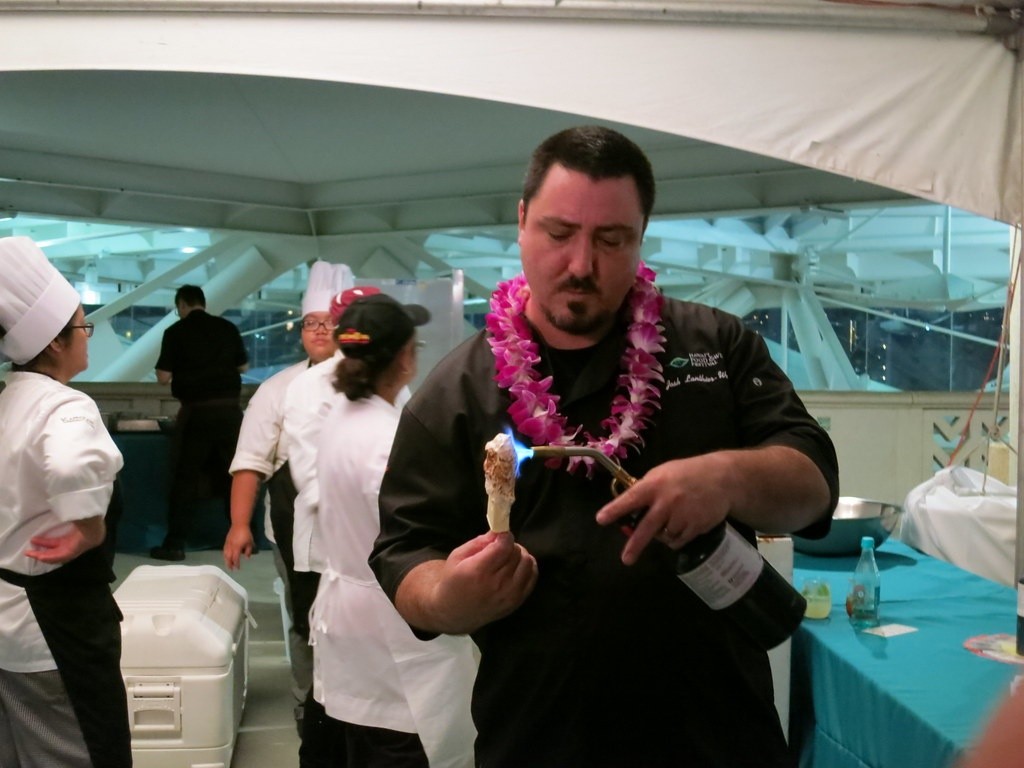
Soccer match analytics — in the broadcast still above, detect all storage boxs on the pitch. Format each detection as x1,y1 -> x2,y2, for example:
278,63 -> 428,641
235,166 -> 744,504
111,564 -> 260,768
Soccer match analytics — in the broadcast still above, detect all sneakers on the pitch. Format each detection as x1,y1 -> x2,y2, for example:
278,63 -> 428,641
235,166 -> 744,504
297,716 -> 304,738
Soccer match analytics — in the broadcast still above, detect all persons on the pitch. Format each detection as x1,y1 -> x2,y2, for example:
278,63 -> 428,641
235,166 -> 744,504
148,284 -> 262,562
221,259 -> 360,740
2,234 -> 132,768
297,288 -> 481,767
286,285 -> 417,652
365,126 -> 844,767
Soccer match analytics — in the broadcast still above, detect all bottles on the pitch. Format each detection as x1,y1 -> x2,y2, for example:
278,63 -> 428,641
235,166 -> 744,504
642,492 -> 806,650
850,530 -> 879,627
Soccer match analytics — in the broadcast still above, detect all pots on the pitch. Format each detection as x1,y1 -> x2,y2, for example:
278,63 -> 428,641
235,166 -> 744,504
788,496 -> 905,554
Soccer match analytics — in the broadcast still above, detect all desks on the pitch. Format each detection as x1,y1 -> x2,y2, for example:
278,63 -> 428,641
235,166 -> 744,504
787,525 -> 1024,768
104,415 -> 176,550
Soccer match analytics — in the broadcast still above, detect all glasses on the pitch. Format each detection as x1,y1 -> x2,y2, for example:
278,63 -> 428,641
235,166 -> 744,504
405,340 -> 428,355
63,322 -> 95,338
300,320 -> 339,331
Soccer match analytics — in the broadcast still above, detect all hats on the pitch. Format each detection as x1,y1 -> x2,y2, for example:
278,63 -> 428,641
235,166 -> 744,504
334,293 -> 431,354
299,257 -> 356,323
0,236 -> 82,365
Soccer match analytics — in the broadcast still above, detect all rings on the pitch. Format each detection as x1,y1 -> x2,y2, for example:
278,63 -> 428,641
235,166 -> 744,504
663,526 -> 680,540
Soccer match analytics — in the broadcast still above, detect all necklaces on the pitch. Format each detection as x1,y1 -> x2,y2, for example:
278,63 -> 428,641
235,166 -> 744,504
485,257 -> 670,480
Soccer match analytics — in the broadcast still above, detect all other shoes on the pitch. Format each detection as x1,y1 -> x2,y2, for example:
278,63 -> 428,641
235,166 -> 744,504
149,545 -> 185,561
